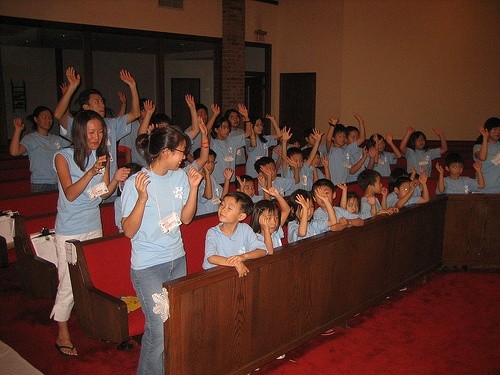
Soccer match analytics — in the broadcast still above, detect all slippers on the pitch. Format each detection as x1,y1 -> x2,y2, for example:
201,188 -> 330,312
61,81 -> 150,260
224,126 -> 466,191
55,341 -> 78,356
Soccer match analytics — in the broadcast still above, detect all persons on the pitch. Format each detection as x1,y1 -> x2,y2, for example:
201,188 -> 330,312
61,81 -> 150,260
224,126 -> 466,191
9,107 -> 73,195
337,181 -> 377,220
121,127 -> 203,375
202,191 -> 269,277
473,118 -> 500,194
50,110 -> 130,356
357,169 -> 399,215
249,187 -> 290,255
55,66 -> 447,216
435,152 -> 485,195
286,188 -> 337,244
311,178 -> 364,233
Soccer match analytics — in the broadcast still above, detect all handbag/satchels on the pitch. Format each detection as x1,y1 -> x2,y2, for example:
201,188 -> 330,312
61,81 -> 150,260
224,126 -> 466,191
0,210 -> 19,250
30,226 -> 58,268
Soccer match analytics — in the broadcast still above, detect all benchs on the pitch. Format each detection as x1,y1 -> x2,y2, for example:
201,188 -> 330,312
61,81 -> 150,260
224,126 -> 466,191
0,141 -> 500,375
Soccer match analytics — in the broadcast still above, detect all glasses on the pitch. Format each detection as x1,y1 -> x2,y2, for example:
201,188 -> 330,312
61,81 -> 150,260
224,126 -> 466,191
175,148 -> 188,155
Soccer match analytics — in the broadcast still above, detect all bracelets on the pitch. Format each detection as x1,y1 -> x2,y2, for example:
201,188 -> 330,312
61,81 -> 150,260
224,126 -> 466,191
245,120 -> 251,123
329,122 -> 335,127
201,142 -> 209,148
